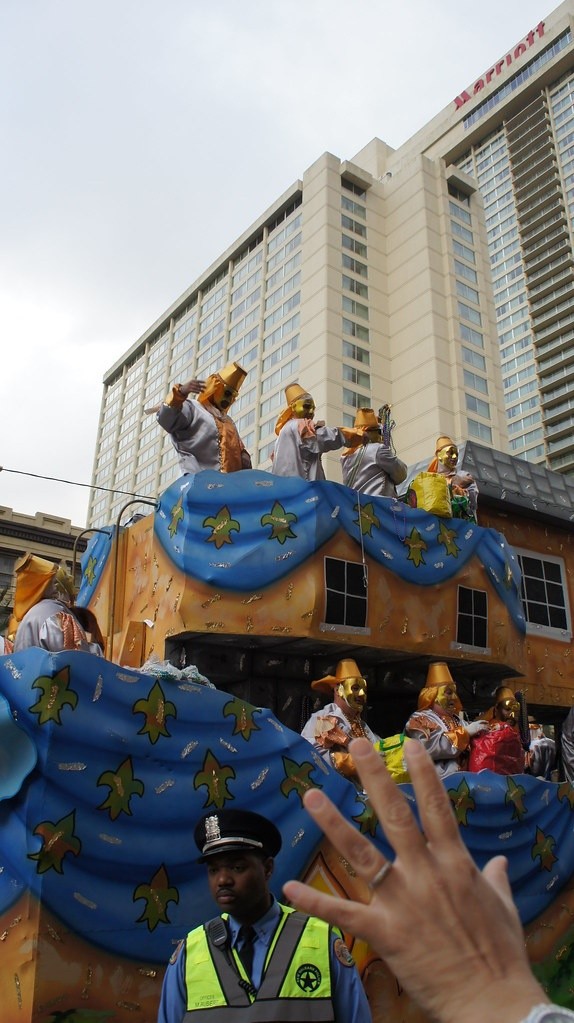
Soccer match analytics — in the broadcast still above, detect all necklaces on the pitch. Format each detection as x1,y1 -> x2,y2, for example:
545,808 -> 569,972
434,712 -> 460,731
342,714 -> 365,739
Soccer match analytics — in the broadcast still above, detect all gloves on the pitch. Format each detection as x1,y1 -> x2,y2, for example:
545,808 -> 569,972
464,720 -> 490,738
452,484 -> 470,498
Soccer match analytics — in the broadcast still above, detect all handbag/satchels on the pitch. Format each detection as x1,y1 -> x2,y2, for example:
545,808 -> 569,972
406,471 -> 453,519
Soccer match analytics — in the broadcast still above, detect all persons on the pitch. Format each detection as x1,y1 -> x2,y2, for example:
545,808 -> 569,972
13,552 -> 91,653
156,362 -> 478,524
301,659 -> 574,777
280,737 -> 574,1023
158,809 -> 371,1023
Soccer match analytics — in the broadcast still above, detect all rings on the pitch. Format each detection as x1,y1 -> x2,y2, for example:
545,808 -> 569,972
366,862 -> 394,891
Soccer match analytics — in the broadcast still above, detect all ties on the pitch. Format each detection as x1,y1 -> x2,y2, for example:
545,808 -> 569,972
239,925 -> 255,990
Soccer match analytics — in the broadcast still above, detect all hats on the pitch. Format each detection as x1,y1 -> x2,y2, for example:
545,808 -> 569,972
217,361 -> 248,393
354,407 -> 380,431
284,383 -> 309,406
426,661 -> 456,689
335,658 -> 363,679
194,807 -> 282,868
14,552 -> 57,575
495,686 -> 516,706
435,437 -> 457,456
